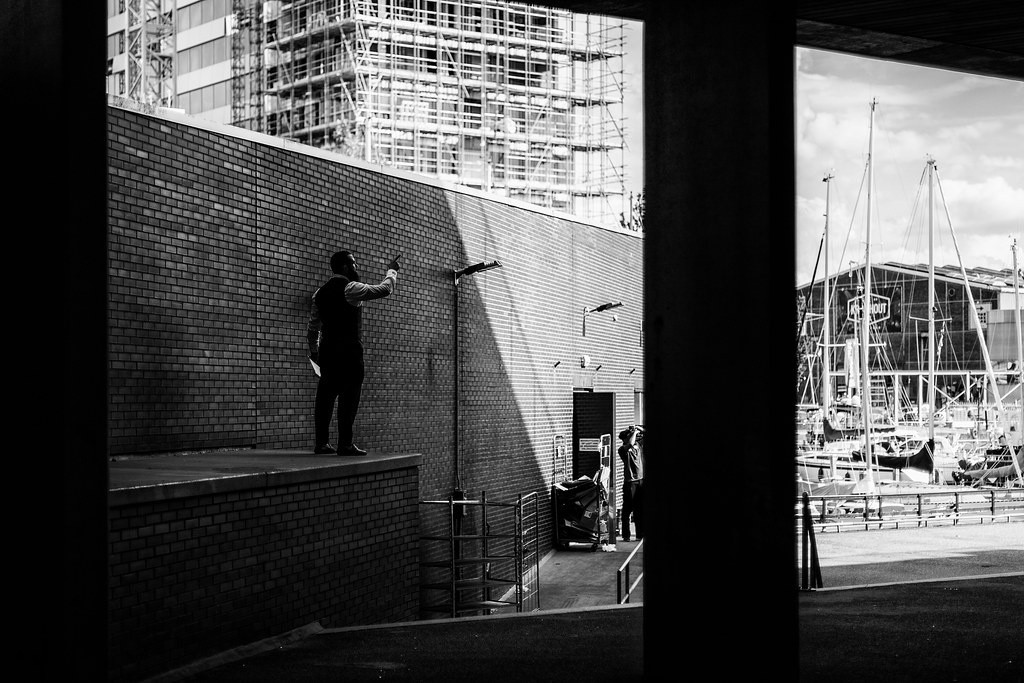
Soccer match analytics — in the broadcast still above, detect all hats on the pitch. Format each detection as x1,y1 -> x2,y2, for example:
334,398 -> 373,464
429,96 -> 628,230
618,429 -> 634,439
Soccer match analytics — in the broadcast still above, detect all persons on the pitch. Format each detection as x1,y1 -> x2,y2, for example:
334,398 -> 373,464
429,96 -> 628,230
307,249 -> 400,456
617,426 -> 646,542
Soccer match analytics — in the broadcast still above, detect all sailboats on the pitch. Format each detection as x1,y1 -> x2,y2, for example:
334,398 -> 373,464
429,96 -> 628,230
797,96 -> 1024,522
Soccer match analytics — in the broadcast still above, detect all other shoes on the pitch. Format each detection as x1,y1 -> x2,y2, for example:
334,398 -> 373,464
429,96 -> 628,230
624,538 -> 630,542
636,537 -> 642,541
337,442 -> 367,456
313,442 -> 336,454
